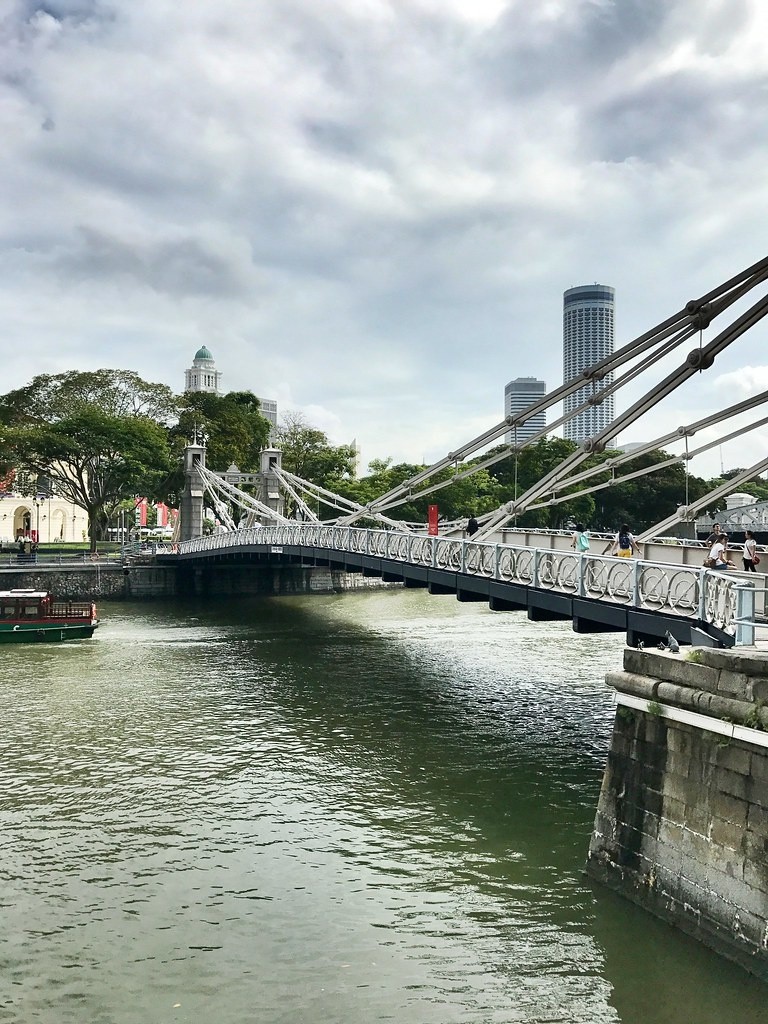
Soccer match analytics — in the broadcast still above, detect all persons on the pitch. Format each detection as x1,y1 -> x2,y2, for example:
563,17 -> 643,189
466,515 -> 478,537
611,524 -> 643,558
572,524 -> 587,553
705,522 -> 756,572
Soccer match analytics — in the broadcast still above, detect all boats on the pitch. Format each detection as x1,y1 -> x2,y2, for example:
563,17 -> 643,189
0,589 -> 100,643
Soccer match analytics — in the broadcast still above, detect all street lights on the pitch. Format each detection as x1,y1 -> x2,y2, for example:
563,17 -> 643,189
32,497 -> 44,543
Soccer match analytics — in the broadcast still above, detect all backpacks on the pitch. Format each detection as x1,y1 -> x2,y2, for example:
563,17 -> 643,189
579,534 -> 590,550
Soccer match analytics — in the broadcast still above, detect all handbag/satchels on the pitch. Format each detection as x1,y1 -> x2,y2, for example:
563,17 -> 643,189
703,558 -> 716,568
619,532 -> 630,549
752,554 -> 761,565
